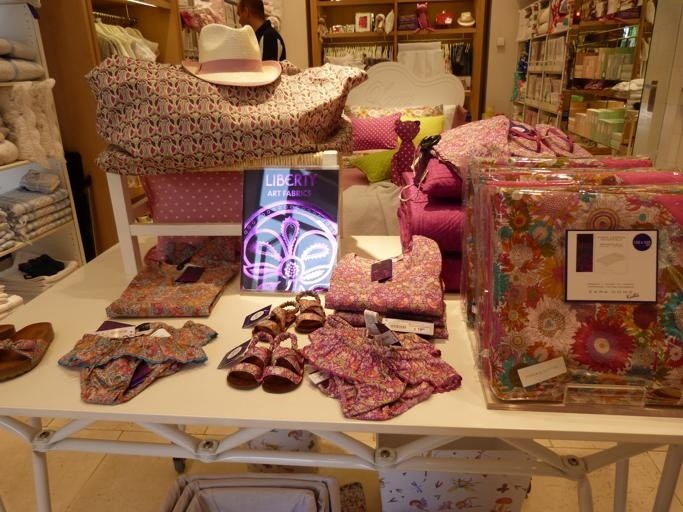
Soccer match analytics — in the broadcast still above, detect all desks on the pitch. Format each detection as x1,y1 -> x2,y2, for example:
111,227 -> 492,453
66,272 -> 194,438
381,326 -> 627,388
0,236 -> 683,510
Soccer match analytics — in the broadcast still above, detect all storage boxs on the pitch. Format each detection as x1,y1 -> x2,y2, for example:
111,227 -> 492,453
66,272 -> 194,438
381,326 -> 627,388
373,431 -> 535,511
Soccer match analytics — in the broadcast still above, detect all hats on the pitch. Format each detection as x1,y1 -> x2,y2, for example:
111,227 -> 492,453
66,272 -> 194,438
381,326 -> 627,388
181,23 -> 283,87
456,10 -> 475,27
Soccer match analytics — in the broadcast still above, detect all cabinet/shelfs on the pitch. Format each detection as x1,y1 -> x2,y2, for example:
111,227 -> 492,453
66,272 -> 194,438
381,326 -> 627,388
0,0 -> 88,320
307,0 -> 487,127
33,0 -> 249,255
509,0 -> 655,158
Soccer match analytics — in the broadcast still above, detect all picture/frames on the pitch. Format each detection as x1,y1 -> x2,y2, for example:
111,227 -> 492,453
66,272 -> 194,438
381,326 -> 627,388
353,12 -> 371,33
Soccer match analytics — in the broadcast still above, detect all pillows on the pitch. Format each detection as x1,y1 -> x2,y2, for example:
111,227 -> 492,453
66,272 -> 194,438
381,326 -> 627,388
30,76 -> 66,166
0,81 -> 50,171
341,105 -> 464,202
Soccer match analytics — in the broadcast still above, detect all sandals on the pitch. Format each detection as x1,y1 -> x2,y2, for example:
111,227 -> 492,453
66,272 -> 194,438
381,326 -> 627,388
295,289 -> 326,333
252,301 -> 299,336
226,331 -> 305,394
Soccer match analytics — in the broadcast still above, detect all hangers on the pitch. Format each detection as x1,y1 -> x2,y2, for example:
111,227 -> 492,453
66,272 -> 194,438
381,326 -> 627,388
323,41 -> 392,63
439,39 -> 470,53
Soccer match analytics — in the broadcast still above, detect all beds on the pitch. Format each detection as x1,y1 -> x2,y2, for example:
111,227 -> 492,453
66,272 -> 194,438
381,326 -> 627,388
336,62 -> 470,238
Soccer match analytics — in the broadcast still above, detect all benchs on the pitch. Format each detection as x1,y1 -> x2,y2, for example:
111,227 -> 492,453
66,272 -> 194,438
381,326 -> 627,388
394,169 -> 465,292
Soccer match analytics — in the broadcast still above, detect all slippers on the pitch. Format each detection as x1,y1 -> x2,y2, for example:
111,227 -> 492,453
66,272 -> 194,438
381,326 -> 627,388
0,322 -> 55,382
507,120 -> 557,159
535,120 -> 595,159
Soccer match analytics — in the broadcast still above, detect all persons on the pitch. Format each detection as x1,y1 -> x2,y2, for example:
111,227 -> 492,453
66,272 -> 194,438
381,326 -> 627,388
237,1 -> 286,62
